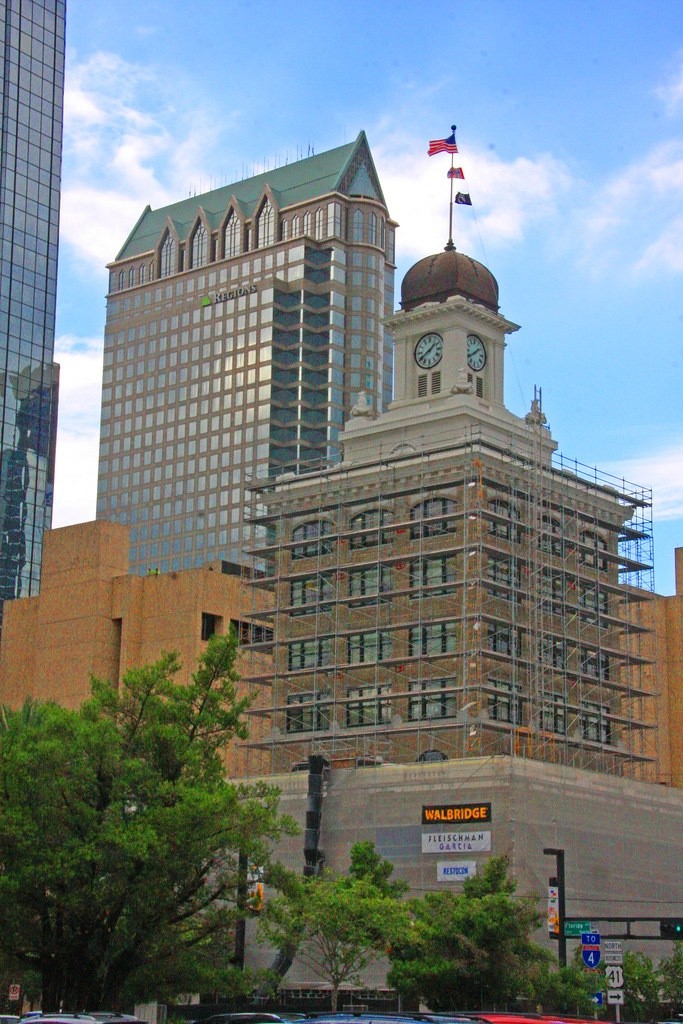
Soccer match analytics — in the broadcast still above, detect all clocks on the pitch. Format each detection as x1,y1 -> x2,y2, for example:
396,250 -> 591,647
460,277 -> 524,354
414,332 -> 443,369
466,334 -> 486,371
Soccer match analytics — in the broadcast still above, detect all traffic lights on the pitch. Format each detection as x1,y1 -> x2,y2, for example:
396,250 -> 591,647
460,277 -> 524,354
660,924 -> 683,935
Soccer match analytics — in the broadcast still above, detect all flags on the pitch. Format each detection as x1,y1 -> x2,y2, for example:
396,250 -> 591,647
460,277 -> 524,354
447,167 -> 464,179
428,134 -> 458,157
455,192 -> 472,206
